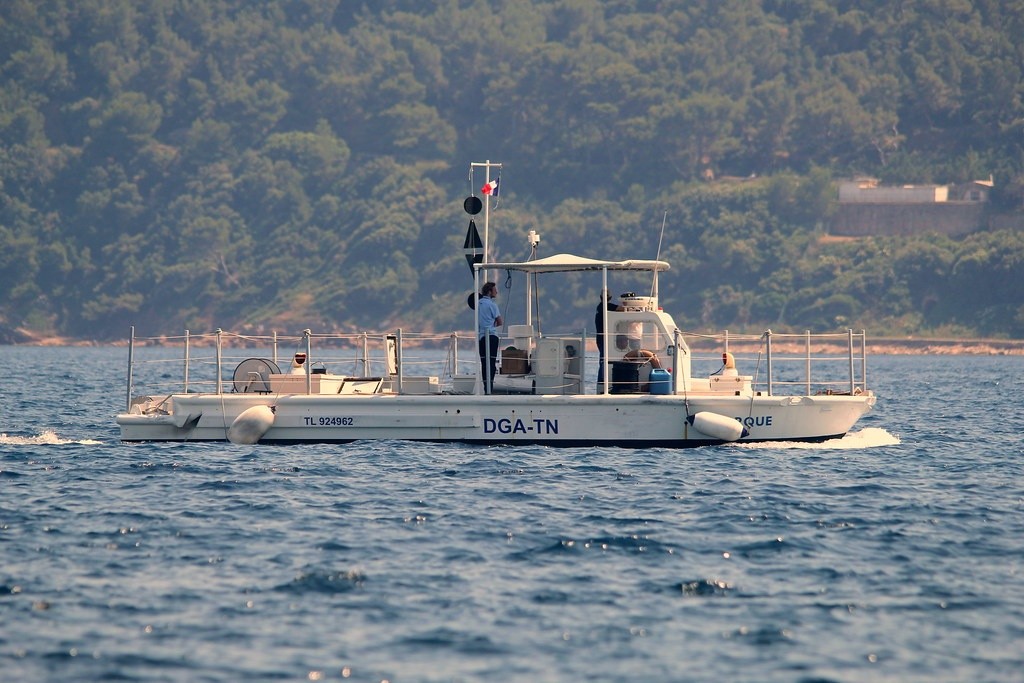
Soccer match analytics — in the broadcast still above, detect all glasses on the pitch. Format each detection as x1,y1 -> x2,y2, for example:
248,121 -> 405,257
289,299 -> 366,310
621,293 -> 635,297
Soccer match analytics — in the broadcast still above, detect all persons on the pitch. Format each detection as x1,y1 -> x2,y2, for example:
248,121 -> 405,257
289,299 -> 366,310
610,291 -> 642,394
477,282 -> 503,395
595,289 -> 627,394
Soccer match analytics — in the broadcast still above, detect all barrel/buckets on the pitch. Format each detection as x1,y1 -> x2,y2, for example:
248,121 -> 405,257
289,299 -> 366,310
649,369 -> 672,394
611,362 -> 639,393
502,349 -> 525,374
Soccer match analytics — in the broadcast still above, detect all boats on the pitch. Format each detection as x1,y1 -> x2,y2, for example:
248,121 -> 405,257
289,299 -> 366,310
116,159 -> 877,450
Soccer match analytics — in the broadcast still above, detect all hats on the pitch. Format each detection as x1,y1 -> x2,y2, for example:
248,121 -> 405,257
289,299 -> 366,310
600,288 -> 611,298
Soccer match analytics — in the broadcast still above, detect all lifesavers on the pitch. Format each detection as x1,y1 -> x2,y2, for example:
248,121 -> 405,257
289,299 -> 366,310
620,349 -> 663,393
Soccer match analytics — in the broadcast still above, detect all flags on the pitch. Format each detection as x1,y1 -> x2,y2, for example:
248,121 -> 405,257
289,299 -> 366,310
481,176 -> 498,196
